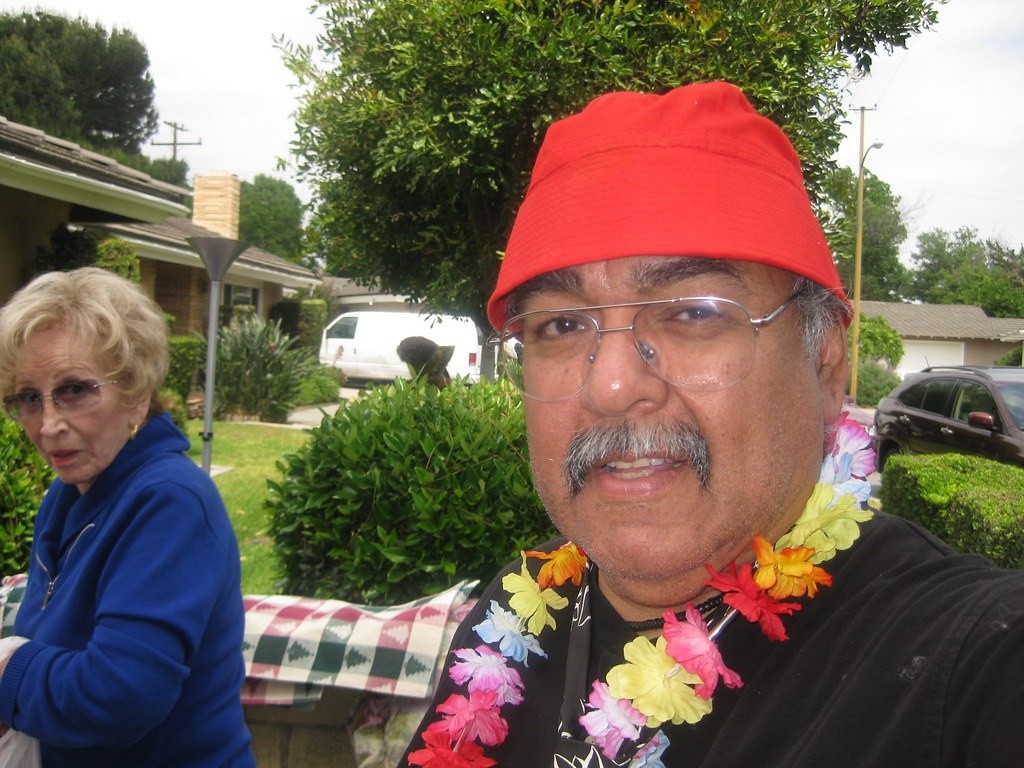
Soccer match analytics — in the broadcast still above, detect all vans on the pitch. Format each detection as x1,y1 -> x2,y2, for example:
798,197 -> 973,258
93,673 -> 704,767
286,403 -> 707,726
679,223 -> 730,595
317,310 -> 524,390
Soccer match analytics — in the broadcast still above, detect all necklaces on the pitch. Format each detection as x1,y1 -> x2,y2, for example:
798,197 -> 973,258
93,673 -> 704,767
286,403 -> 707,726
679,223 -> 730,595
400,409 -> 882,768
619,590 -> 733,629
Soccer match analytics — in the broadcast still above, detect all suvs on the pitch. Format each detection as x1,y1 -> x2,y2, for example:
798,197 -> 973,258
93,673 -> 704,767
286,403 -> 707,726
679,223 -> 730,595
873,364 -> 1024,471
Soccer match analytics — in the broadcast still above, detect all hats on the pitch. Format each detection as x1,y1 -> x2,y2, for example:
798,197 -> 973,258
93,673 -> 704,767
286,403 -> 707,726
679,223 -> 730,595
484,80 -> 853,334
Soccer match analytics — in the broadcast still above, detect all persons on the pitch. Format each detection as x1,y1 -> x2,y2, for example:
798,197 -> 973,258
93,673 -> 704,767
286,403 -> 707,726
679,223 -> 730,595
0,264 -> 253,768
393,83 -> 1024,768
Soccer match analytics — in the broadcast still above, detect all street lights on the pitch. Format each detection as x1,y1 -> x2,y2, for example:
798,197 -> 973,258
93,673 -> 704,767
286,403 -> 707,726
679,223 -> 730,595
849,141 -> 883,406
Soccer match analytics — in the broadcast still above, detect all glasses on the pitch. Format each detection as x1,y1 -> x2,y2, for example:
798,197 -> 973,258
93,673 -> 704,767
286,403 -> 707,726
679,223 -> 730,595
2,379 -> 129,424
486,288 -> 804,401
